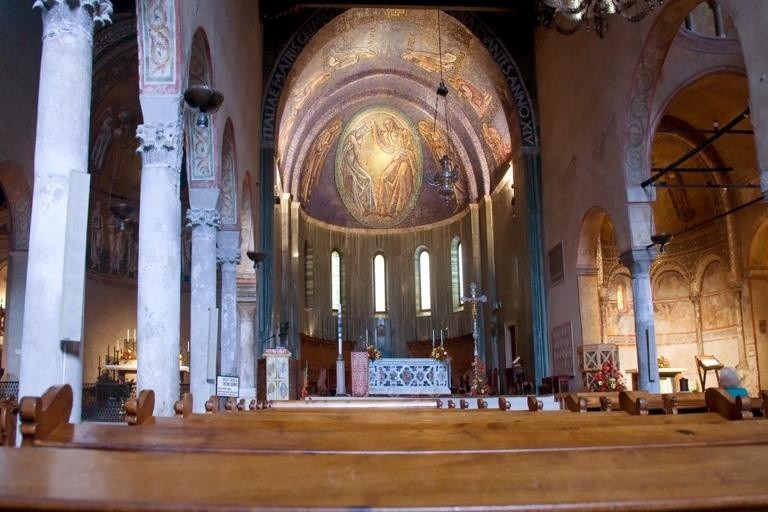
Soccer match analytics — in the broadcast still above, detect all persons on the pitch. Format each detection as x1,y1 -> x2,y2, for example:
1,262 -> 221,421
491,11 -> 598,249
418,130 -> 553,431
328,45 -> 378,70
399,50 -> 457,74
480,118 -> 511,166
417,119 -> 468,214
301,122 -> 340,201
447,78 -> 487,112
276,116 -> 295,168
719,367 -> 748,398
289,72 -> 333,112
342,122 -> 377,216
369,116 -> 418,218
85,104 -> 138,281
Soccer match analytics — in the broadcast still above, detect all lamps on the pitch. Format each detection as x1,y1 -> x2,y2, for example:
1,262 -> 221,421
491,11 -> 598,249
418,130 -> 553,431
423,8 -> 462,207
246,180 -> 266,267
180,80 -> 224,133
634,13 -> 675,252
108,113 -> 138,229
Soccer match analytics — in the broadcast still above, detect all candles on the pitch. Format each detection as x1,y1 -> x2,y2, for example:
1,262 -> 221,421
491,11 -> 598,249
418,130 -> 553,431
133,328 -> 136,342
117,340 -> 119,351
127,329 -> 129,342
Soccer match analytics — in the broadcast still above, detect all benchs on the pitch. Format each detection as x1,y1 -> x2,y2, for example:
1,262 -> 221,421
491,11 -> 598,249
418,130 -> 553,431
0,387 -> 768,511
